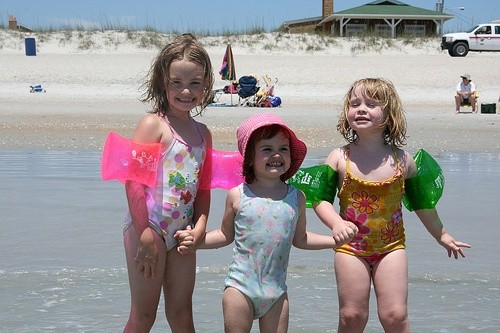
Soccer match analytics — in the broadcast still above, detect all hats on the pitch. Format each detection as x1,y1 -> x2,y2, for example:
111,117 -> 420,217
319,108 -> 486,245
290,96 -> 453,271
460,73 -> 471,82
236,113 -> 308,182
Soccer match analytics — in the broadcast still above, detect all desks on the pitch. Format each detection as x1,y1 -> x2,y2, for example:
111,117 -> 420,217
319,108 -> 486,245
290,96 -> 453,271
214,92 -> 253,107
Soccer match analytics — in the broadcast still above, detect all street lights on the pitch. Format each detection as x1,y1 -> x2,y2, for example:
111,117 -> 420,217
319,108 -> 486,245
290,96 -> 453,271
445,6 -> 465,13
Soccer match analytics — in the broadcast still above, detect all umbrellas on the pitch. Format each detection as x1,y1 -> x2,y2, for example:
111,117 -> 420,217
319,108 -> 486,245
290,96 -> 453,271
219,42 -> 236,106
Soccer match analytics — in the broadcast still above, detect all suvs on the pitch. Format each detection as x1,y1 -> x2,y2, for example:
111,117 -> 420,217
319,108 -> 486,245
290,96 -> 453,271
440,20 -> 500,58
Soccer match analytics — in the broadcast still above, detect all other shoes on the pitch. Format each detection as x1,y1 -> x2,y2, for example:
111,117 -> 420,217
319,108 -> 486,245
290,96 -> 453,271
472,109 -> 476,113
456,109 -> 460,113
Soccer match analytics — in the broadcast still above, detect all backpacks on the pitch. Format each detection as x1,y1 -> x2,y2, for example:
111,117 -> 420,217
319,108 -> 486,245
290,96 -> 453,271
238,75 -> 258,98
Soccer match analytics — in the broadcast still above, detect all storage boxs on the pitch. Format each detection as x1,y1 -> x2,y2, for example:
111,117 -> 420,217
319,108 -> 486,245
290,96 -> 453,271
481,103 -> 496,113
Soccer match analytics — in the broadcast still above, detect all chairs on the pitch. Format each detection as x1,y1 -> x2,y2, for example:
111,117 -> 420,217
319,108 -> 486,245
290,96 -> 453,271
238,76 -> 260,107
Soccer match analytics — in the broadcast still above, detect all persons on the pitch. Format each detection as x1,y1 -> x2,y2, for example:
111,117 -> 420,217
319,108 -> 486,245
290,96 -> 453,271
311,78 -> 471,333
123,33 -> 214,333
455,73 -> 476,114
177,113 -> 355,333
